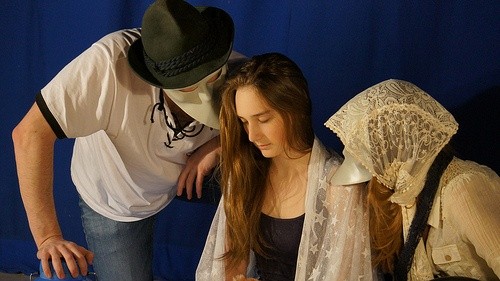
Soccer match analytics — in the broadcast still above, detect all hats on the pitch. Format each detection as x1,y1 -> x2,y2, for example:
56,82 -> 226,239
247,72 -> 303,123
330,145 -> 377,187
128,0 -> 235,88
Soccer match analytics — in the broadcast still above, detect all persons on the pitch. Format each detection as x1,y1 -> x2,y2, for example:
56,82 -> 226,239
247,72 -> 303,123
324,79 -> 500,281
13,0 -> 259,280
190,54 -> 376,281
